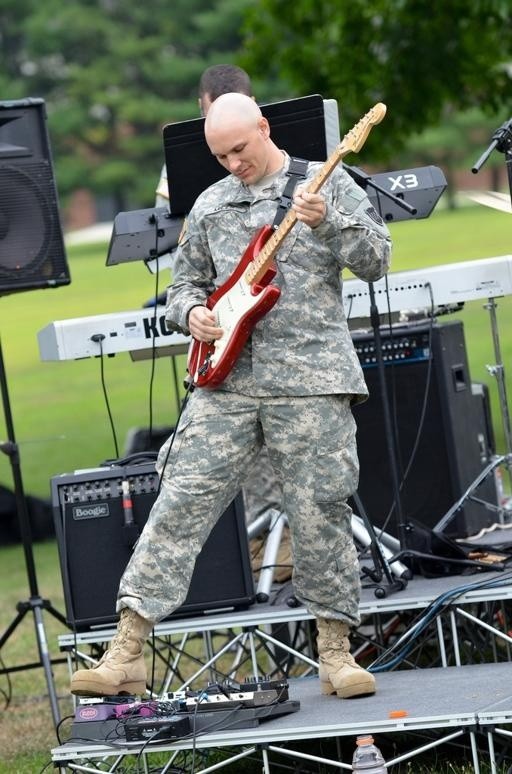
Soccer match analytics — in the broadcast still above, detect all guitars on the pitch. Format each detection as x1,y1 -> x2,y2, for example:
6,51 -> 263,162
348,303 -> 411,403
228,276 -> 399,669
186,104 -> 389,389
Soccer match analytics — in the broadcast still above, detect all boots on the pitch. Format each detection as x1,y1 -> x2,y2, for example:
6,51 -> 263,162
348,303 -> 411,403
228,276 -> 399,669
67,604 -> 158,699
314,615 -> 379,699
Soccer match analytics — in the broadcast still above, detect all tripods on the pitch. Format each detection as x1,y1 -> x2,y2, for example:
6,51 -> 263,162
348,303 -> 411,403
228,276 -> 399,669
1,342 -> 107,741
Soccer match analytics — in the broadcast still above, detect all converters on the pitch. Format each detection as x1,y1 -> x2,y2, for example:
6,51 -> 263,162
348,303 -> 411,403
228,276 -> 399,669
123,715 -> 189,741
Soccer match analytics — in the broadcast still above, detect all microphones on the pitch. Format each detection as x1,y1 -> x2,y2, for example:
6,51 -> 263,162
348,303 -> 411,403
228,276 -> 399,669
121,478 -> 138,550
400,305 -> 460,322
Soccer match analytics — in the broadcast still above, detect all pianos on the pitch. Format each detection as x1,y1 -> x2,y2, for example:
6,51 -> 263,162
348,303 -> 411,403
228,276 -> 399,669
35,256 -> 512,363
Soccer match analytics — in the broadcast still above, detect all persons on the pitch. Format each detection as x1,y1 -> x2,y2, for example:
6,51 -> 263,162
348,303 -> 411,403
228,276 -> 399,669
68,89 -> 397,698
155,63 -> 297,586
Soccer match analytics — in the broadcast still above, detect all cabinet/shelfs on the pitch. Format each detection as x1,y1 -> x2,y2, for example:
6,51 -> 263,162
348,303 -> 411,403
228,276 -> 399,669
49,565 -> 512,774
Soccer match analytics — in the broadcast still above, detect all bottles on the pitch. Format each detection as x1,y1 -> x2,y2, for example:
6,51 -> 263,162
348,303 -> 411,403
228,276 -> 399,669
352,735 -> 388,774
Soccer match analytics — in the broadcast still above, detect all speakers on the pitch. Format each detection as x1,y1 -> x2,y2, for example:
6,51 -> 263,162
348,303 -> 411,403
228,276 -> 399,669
0,95 -> 70,297
346,320 -> 499,552
49,458 -> 256,631
